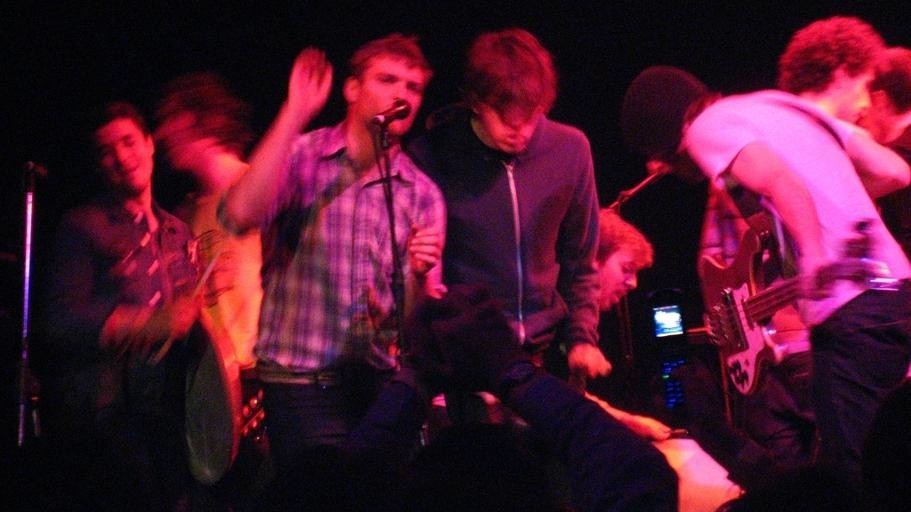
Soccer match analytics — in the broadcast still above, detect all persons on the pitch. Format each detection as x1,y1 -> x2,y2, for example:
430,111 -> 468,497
619,17 -> 911,511
51,28 -> 679,512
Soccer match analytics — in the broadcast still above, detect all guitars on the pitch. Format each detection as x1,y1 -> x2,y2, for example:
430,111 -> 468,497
703,219 -> 901,396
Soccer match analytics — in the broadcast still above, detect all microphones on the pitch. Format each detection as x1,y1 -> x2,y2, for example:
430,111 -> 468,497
370,98 -> 412,129
21,160 -> 50,177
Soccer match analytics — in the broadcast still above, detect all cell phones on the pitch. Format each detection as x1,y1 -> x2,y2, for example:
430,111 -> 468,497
647,286 -> 692,413
